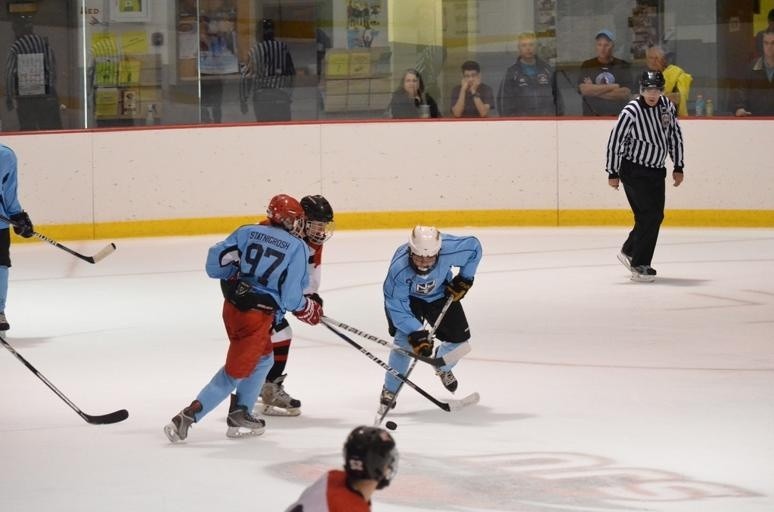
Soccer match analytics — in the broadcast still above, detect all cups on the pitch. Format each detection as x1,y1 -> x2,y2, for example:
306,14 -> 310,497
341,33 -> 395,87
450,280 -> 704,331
418,105 -> 430,119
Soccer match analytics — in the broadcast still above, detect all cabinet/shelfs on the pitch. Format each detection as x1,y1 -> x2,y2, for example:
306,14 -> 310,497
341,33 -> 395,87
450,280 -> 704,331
319,46 -> 392,114
90,52 -> 163,121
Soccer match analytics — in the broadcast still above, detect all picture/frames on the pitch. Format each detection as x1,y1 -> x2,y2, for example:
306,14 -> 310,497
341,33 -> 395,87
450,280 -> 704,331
107,0 -> 151,24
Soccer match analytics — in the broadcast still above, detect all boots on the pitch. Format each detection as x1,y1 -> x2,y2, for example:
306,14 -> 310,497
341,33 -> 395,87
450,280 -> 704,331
225,393 -> 266,439
162,400 -> 204,443
256,372 -> 301,417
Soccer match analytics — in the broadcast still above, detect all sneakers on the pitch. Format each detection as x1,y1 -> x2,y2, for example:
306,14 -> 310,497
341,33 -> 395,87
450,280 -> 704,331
617,244 -> 633,271
433,365 -> 458,394
0,311 -> 10,338
630,263 -> 657,284
378,387 -> 397,415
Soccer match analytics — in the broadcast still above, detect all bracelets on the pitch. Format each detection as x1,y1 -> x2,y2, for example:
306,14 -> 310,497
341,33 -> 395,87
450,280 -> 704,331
472,92 -> 481,98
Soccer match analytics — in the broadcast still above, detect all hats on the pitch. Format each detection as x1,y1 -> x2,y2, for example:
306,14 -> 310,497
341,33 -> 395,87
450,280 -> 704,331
593,29 -> 616,43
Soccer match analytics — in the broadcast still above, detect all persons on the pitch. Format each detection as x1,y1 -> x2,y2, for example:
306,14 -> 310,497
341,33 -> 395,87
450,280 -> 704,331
192,15 -> 224,124
163,194 -> 325,444
385,68 -> 443,119
381,226 -> 482,407
282,426 -> 399,512
576,30 -> 632,117
3,15 -> 62,130
252,196 -> 334,417
628,44 -> 692,118
730,31 -> 772,117
449,61 -> 494,119
0,145 -> 32,331
236,18 -> 296,122
604,69 -> 686,278
750,9 -> 772,64
495,31 -> 565,117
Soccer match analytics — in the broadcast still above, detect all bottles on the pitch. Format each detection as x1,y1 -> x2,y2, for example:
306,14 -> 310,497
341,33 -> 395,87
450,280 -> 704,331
695,95 -> 704,116
705,96 -> 713,115
145,104 -> 160,125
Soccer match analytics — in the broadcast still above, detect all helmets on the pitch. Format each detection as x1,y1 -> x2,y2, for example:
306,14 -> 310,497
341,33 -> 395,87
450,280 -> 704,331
408,225 -> 443,272
11,15 -> 33,38
638,70 -> 665,97
342,425 -> 398,490
299,194 -> 333,237
254,18 -> 274,42
264,194 -> 306,233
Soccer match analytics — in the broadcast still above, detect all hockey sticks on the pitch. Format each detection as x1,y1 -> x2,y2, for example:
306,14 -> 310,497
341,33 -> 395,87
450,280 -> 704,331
320,295 -> 480,429
0,338 -> 129,424
0,214 -> 116,263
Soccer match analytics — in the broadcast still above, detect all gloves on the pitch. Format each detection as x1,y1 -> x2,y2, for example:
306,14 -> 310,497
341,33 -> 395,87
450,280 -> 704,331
8,212 -> 33,238
407,330 -> 434,357
443,274 -> 472,302
291,293 -> 323,326
